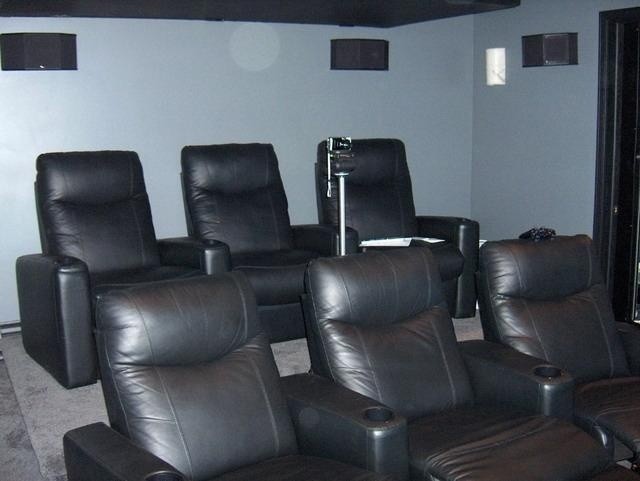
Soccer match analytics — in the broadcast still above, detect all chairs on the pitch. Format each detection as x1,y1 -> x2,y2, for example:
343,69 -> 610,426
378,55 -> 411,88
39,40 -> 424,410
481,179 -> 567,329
180,139 -> 360,344
15,149 -> 231,389
299,242 -> 615,480
315,134 -> 480,320
63,270 -> 411,481
479,233 -> 640,468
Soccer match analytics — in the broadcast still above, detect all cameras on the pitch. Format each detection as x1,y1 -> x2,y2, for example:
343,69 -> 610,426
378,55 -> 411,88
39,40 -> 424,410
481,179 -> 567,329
326,136 -> 353,154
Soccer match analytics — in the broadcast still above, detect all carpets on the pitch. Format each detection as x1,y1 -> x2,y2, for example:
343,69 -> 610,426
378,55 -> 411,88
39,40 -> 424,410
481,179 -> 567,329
0,314 -> 485,481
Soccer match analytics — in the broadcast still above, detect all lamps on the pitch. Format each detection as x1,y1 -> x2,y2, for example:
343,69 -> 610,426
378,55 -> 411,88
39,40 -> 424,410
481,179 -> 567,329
484,47 -> 507,86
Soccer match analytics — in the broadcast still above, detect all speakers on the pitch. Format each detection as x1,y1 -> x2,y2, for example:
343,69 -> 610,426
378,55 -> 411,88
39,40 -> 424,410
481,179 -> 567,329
521,32 -> 578,68
0,33 -> 77,71
330,38 -> 389,71
